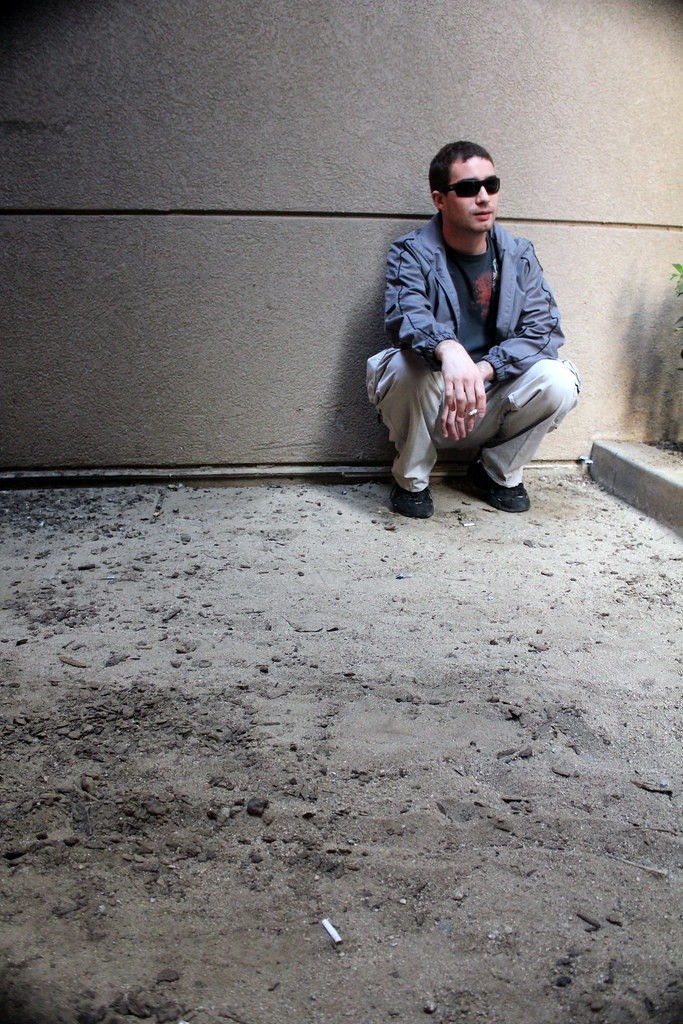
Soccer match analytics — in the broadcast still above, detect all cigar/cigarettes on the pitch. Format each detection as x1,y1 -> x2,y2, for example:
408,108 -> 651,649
468,409 -> 478,416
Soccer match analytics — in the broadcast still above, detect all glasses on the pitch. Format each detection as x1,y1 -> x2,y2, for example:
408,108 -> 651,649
444,176 -> 500,197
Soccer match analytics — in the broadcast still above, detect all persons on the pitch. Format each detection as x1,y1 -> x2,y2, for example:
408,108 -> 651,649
365,140 -> 582,518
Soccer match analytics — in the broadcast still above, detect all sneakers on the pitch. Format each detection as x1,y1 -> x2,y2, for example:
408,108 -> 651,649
475,472 -> 529,512
390,478 -> 433,518
